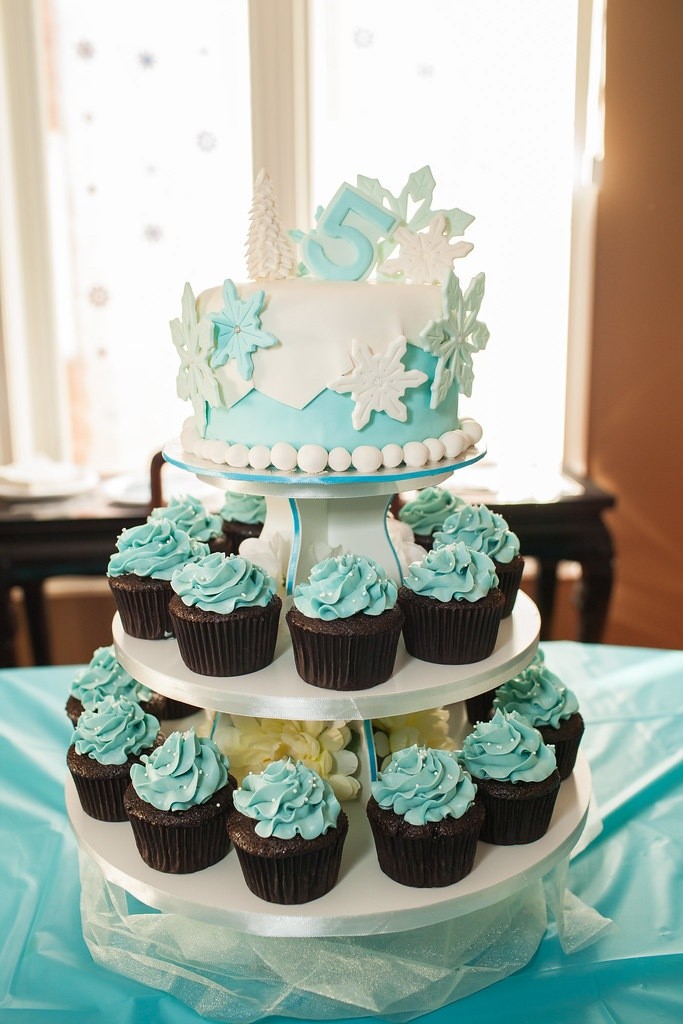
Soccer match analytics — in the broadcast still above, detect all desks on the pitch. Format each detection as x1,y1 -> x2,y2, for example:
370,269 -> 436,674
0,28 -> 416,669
0,640 -> 683,1024
0,459 -> 616,671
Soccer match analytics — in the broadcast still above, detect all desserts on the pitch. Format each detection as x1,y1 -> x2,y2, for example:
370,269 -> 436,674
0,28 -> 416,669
107,492 -> 526,691
65,645 -> 586,904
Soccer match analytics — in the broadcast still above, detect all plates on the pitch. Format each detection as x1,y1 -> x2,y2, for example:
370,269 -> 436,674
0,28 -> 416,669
0,464 -> 98,499
105,475 -> 164,506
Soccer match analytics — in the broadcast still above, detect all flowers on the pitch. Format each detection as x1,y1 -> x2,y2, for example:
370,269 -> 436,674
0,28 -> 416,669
239,530 -> 291,598
373,706 -> 456,773
213,713 -> 363,801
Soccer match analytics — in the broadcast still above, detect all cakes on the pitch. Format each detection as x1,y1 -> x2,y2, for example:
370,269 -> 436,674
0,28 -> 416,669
170,164 -> 490,476
